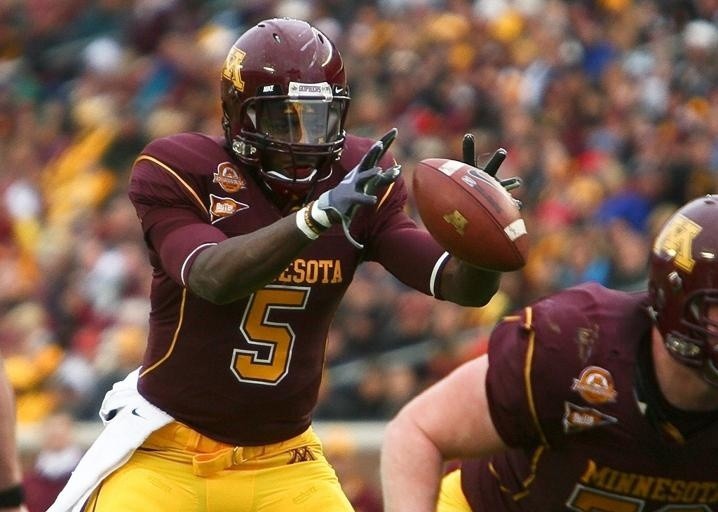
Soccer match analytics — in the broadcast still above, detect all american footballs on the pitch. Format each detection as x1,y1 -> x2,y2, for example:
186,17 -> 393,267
413,158 -> 528,273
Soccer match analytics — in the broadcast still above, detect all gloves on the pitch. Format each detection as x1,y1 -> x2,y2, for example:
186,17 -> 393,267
453,130 -> 527,215
315,128 -> 404,252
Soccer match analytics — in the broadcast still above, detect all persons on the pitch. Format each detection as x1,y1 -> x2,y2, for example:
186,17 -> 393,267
2,1 -> 718,512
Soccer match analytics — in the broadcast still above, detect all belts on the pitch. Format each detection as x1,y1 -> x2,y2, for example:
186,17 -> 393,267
141,412 -> 318,481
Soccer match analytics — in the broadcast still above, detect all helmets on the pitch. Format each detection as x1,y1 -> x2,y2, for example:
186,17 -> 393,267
643,191 -> 718,368
216,14 -> 353,166
